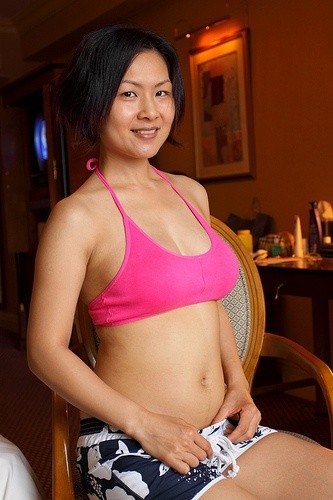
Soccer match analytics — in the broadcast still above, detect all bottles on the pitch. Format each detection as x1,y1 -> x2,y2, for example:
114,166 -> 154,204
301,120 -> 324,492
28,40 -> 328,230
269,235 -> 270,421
237,229 -> 253,253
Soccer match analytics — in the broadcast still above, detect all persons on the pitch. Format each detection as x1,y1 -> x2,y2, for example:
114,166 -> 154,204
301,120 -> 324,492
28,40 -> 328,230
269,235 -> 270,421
26,24 -> 333,500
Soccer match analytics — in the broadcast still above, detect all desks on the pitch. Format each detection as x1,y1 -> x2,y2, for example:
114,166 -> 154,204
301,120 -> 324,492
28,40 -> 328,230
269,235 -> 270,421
256,253 -> 333,415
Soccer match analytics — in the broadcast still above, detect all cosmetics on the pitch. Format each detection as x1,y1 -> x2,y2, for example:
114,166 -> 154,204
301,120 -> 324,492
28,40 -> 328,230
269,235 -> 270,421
293,214 -> 304,258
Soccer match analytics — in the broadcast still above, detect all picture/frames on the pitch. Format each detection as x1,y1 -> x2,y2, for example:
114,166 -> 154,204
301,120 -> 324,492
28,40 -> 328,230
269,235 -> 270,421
189,27 -> 257,182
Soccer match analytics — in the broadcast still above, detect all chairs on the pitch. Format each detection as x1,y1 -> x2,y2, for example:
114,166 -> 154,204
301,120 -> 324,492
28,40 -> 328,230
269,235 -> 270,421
52,215 -> 333,500
16,251 -> 30,351
